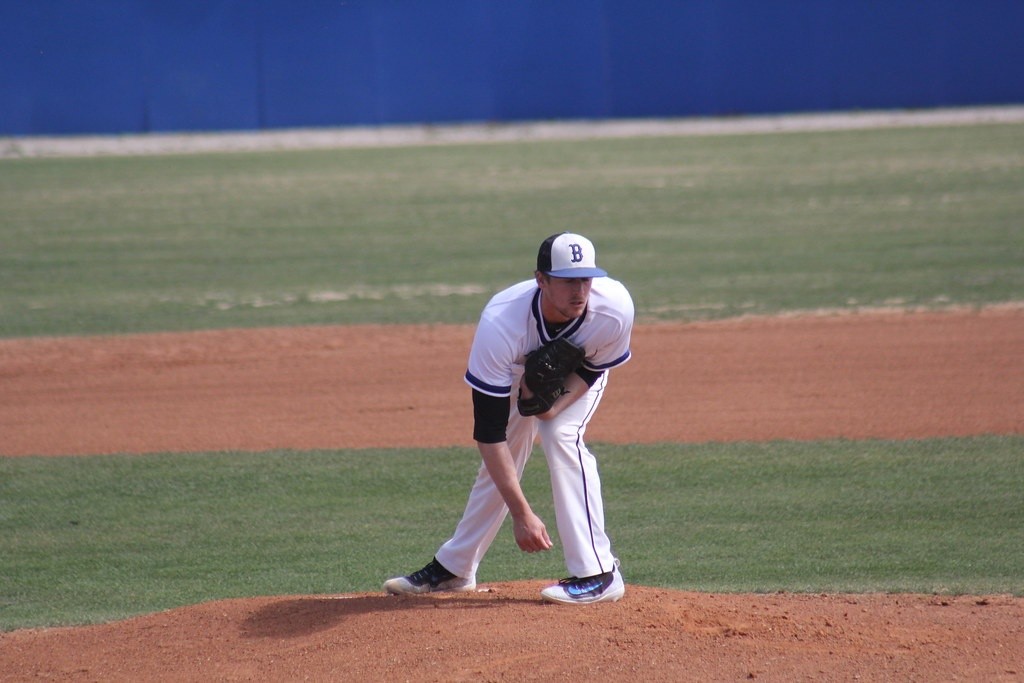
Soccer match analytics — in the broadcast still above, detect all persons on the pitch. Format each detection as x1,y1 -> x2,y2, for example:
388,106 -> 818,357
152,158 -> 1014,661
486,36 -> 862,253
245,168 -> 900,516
383,231 -> 635,605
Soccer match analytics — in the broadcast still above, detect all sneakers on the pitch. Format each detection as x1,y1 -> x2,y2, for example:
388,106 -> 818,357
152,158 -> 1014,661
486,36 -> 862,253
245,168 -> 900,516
541,562 -> 624,605
386,558 -> 476,595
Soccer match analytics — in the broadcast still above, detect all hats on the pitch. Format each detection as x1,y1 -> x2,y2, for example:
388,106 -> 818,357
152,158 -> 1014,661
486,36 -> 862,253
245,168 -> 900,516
537,231 -> 607,278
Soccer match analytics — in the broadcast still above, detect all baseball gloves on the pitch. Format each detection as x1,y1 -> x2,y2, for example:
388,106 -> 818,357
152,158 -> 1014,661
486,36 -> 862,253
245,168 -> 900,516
517,338 -> 585,417
